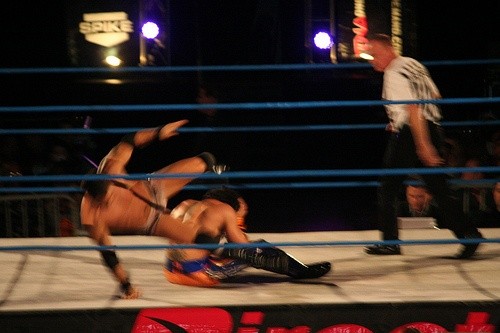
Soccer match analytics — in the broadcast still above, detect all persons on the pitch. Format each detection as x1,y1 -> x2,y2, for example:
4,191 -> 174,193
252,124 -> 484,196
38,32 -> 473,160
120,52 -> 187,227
197,83 -> 227,127
163,187 -> 330,288
81,120 -> 230,299
439,138 -> 500,228
398,185 -> 437,217
363,33 -> 483,260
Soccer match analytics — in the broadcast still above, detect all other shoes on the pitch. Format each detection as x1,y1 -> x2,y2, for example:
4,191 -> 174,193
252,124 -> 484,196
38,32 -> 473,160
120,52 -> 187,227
455,233 -> 483,257
302,262 -> 330,278
365,244 -> 400,255
